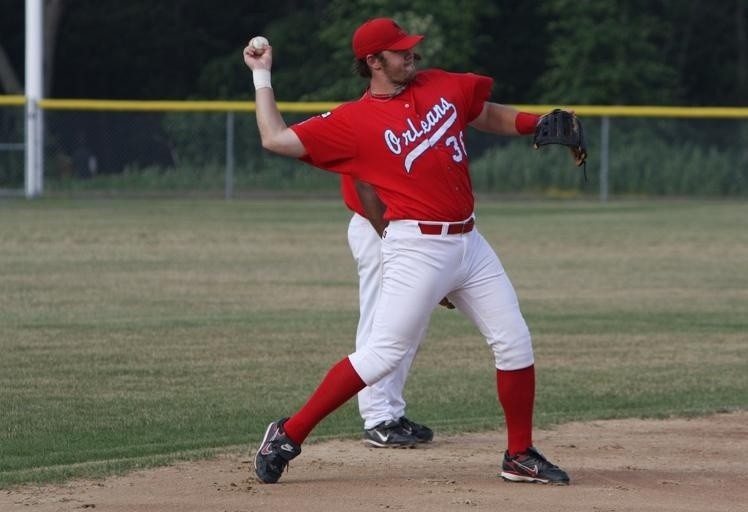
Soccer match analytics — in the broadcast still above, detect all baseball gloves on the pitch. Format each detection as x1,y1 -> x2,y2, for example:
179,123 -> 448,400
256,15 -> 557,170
532,109 -> 588,167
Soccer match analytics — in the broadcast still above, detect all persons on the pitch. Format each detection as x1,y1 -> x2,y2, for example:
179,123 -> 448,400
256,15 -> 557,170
241,19 -> 588,484
345,48 -> 460,450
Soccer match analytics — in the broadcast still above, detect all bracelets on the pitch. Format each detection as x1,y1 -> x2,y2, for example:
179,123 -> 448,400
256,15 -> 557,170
252,67 -> 273,92
516,109 -> 541,135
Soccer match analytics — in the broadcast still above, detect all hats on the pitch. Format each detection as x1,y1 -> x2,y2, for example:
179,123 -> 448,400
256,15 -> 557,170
353,15 -> 425,62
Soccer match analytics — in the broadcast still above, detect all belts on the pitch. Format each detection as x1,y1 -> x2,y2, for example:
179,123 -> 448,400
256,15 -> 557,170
419,216 -> 475,235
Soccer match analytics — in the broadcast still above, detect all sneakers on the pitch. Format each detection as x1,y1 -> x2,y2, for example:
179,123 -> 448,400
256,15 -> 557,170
252,416 -> 302,485
362,414 -> 434,449
497,444 -> 570,487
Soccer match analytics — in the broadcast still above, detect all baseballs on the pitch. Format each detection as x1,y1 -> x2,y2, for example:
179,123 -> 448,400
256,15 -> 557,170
251,36 -> 270,56
